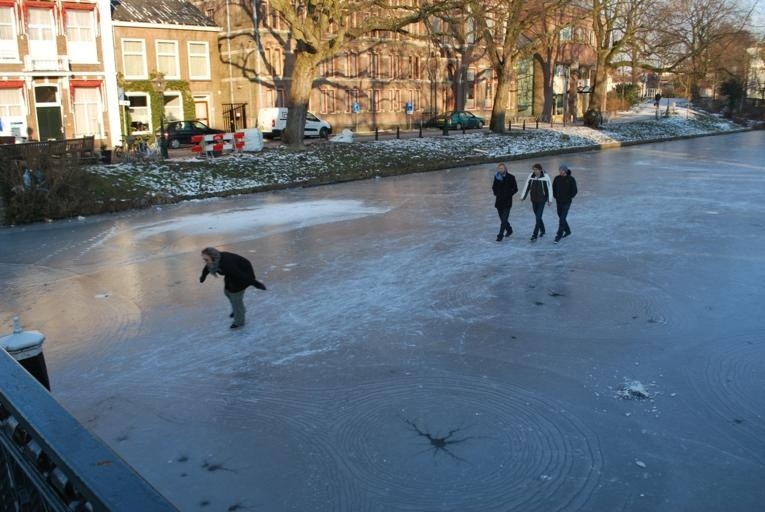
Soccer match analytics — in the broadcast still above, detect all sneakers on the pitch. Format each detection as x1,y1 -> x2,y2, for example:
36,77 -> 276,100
230,312 -> 238,328
496,228 -> 571,243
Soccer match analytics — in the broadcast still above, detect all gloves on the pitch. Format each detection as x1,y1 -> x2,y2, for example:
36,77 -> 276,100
256,282 -> 266,290
200,276 -> 205,283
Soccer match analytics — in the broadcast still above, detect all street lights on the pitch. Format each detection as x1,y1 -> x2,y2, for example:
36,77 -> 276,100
151,73 -> 170,136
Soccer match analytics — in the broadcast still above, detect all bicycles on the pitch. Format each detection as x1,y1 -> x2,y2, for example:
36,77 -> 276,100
114,139 -> 151,160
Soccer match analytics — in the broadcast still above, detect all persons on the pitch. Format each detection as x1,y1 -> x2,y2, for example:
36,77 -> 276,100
552,162 -> 577,244
519,164 -> 553,241
492,163 -> 519,241
198,248 -> 267,329
654,93 -> 661,106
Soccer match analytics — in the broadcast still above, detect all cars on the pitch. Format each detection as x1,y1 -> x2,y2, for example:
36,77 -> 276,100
154,120 -> 230,149
258,107 -> 332,141
434,110 -> 485,130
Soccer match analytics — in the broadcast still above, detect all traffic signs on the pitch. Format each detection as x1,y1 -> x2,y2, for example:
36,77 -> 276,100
406,102 -> 413,114
352,103 -> 361,112
